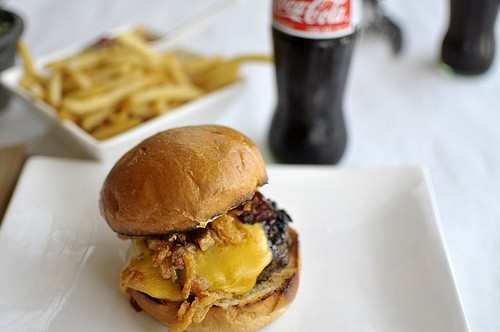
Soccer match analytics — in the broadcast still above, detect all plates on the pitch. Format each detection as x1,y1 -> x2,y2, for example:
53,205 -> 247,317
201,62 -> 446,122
0,155 -> 471,332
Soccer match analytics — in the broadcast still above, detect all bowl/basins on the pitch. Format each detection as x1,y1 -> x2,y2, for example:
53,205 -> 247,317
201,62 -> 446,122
0,9 -> 25,109
0,20 -> 250,164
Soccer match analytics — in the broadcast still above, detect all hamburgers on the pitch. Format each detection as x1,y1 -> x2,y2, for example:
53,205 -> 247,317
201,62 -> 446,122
99,124 -> 302,332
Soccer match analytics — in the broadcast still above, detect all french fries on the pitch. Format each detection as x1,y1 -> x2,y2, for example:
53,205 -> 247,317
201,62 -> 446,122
19,24 -> 276,141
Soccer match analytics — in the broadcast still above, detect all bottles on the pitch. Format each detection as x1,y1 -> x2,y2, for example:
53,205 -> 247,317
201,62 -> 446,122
267,0 -> 361,169
440,0 -> 500,78
